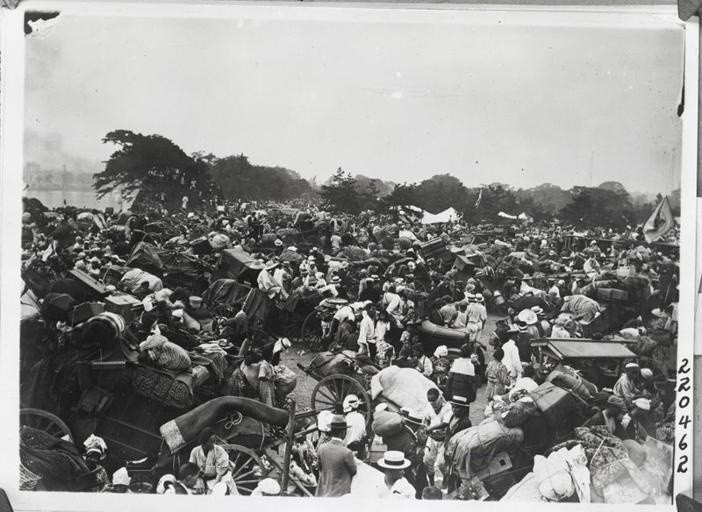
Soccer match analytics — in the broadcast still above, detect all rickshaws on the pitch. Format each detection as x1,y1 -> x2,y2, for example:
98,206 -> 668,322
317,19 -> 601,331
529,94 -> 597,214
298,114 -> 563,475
296,342 -> 422,434
120,416 -> 269,494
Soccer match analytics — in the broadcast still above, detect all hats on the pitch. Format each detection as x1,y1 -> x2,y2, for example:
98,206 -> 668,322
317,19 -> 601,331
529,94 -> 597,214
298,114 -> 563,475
522,274 -> 532,280
264,261 -> 278,270
450,395 -> 469,407
377,450 -> 411,470
274,239 -> 283,246
300,268 -> 341,285
460,284 -> 484,307
518,306 -> 543,333
259,479 -> 280,495
371,274 -> 380,281
326,415 -> 350,431
